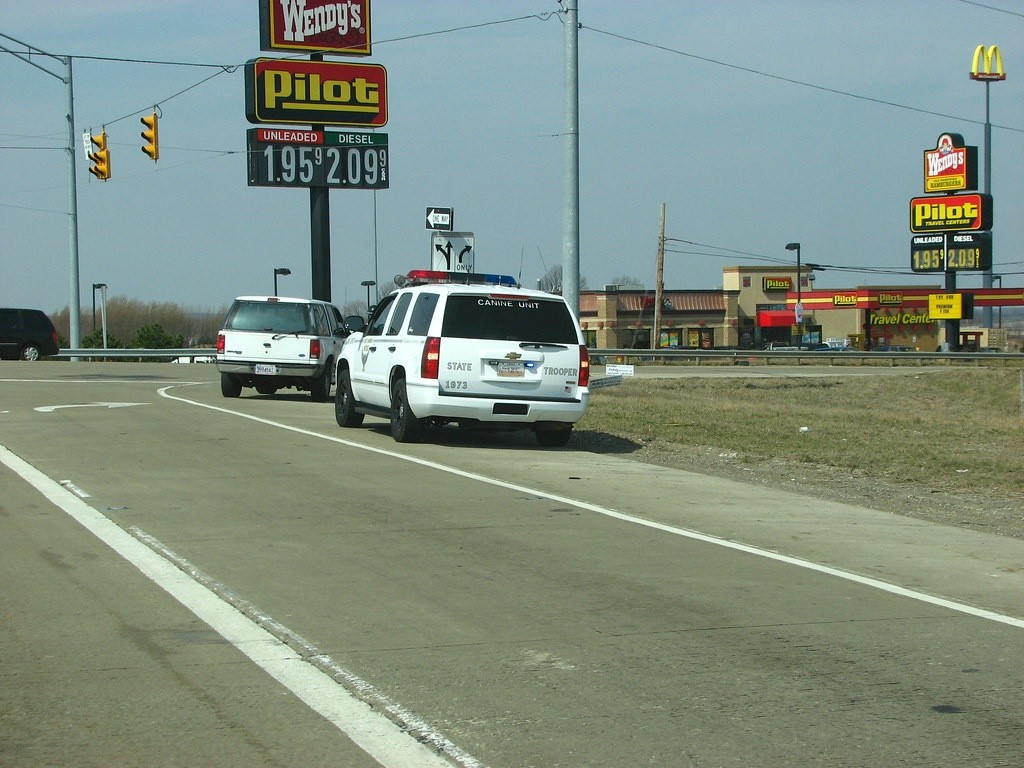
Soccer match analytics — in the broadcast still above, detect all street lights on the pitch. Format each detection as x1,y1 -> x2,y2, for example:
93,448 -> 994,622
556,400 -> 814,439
360,279 -> 375,308
784,242 -> 803,350
274,268 -> 291,297
92,281 -> 108,331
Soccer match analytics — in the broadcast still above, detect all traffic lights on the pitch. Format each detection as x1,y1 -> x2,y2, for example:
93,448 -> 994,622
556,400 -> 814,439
88,133 -> 112,181
140,114 -> 160,165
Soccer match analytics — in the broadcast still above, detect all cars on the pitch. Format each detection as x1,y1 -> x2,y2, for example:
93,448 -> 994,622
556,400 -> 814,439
658,339 -> 1001,360
0,308 -> 59,362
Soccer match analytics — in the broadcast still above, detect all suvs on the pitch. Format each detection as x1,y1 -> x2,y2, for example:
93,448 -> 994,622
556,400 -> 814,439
333,271 -> 590,445
216,295 -> 350,406
